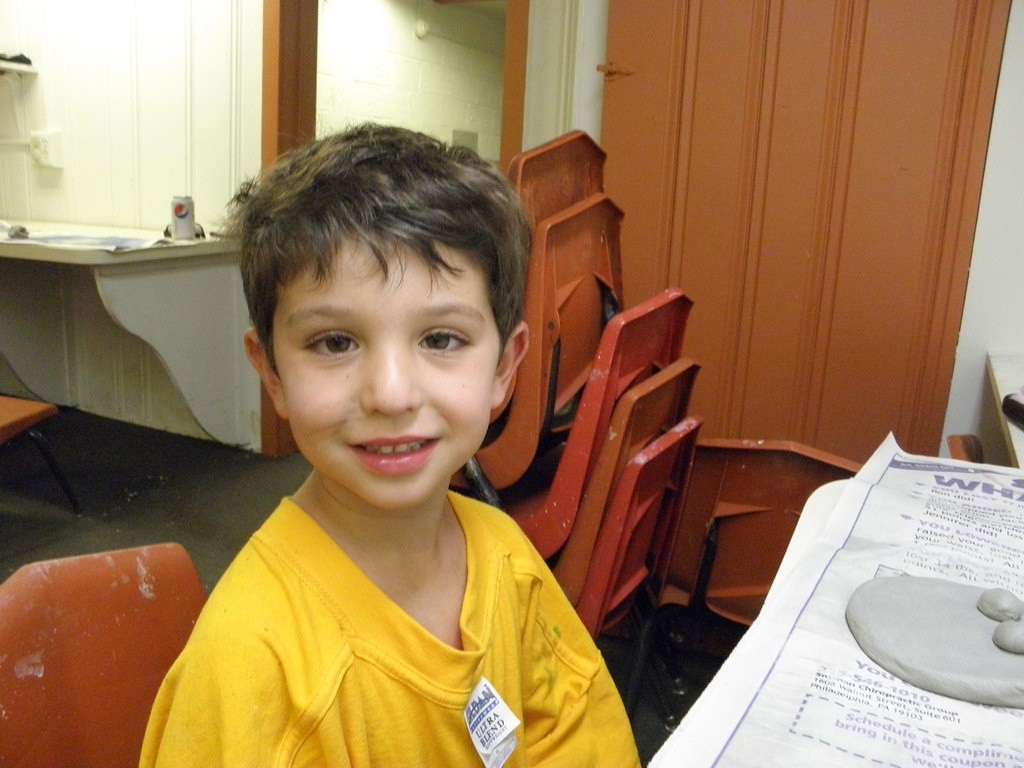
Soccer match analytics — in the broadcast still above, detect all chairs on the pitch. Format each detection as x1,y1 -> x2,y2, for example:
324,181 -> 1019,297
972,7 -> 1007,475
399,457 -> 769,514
0,541 -> 209,768
448,128 -> 706,723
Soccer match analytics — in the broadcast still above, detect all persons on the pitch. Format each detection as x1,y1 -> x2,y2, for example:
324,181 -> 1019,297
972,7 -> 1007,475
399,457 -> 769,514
138,117 -> 643,768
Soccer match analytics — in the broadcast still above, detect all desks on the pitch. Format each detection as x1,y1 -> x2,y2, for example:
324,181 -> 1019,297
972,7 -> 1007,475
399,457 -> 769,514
0,220 -> 264,463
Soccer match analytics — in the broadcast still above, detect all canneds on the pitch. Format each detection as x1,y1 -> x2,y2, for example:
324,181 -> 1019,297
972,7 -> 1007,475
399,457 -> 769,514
170,195 -> 195,240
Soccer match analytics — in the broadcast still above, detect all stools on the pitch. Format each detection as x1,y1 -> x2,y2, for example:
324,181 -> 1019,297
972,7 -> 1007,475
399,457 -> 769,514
0,393 -> 82,515
